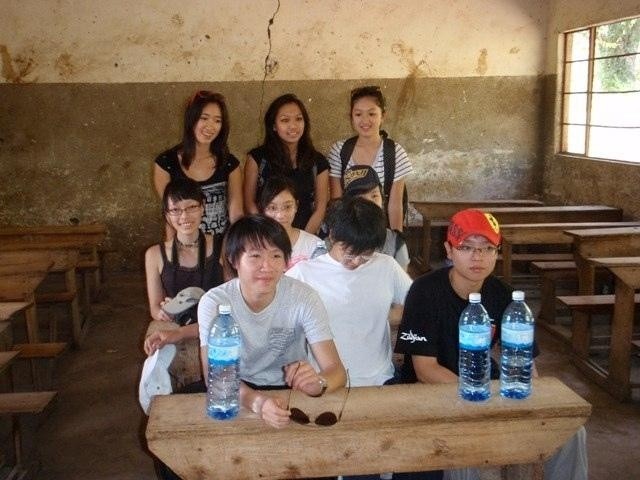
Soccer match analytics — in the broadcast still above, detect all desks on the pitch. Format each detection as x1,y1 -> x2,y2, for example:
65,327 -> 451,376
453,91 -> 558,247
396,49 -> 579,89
0,349 -> 23,480
498,221 -> 640,287
0,251 -> 59,342
145,376 -> 592,480
471,205 -> 624,224
563,227 -> 639,347
34,250 -> 84,350
0,301 -> 34,393
1,223 -> 107,305
588,256 -> 640,402
409,199 -> 544,274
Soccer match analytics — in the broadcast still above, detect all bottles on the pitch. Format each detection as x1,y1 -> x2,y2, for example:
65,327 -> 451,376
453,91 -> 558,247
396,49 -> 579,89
207,304 -> 242,420
457,292 -> 492,401
499,291 -> 535,400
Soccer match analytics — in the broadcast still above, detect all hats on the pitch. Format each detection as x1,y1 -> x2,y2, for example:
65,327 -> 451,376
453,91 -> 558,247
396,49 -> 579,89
447,209 -> 502,246
342,165 -> 380,195
163,285 -> 206,315
138,343 -> 177,417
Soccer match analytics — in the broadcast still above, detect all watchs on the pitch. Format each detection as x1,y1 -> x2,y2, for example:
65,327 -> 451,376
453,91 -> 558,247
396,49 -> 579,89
308,374 -> 328,398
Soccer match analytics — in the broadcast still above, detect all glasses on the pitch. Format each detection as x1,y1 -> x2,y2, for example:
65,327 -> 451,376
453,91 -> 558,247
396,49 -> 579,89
350,85 -> 382,96
166,202 -> 201,215
455,245 -> 501,257
266,204 -> 291,212
190,89 -> 225,108
287,361 -> 350,426
343,254 -> 373,261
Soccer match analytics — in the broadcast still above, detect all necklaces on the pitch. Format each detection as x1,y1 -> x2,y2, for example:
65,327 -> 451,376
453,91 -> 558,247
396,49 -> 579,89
176,238 -> 199,247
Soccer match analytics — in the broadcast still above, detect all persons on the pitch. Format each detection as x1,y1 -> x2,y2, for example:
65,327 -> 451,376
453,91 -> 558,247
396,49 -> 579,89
146,179 -> 236,323
145,199 -> 414,386
197,215 -> 348,431
394,211 -> 587,479
324,165 -> 410,274
254,175 -> 330,271
244,94 -> 330,235
327,86 -> 414,232
153,91 -> 244,238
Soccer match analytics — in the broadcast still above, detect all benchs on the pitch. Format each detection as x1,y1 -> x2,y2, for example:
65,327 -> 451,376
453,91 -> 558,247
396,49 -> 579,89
531,261 -> 607,325
402,222 -> 449,263
496,253 -> 573,279
12,342 -> 68,388
0,391 -> 60,476
556,293 -> 640,350
76,261 -> 103,313
80,239 -> 122,282
31,291 -> 76,342
